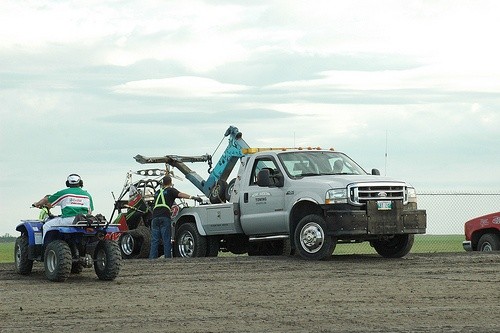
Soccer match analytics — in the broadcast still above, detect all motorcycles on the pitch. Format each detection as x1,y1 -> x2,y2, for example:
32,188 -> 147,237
13,200 -> 123,283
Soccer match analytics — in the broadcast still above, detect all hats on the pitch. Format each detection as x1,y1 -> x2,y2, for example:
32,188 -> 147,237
162,176 -> 172,180
67,175 -> 81,184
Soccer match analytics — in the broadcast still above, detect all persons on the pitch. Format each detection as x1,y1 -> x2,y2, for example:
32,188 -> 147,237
32,174 -> 94,217
149,176 -> 203,260
330,159 -> 344,173
38,194 -> 54,220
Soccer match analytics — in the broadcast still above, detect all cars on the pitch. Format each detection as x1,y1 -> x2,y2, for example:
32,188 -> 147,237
462,212 -> 500,252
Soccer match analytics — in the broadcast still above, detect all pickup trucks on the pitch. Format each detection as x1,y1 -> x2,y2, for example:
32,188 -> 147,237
171,148 -> 428,262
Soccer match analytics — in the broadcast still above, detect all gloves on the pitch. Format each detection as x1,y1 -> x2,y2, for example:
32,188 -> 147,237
191,196 -> 203,203
